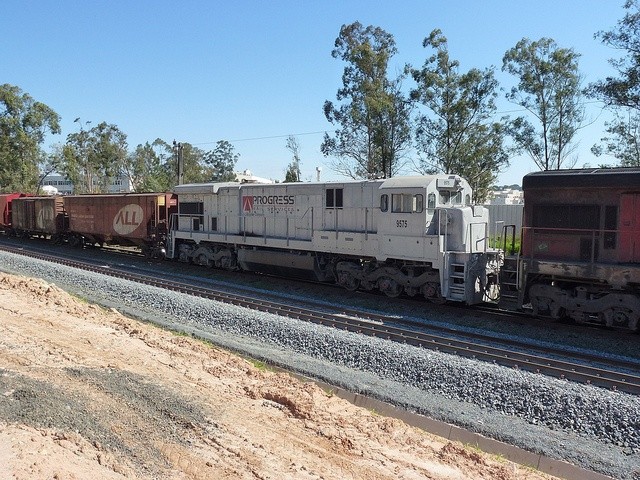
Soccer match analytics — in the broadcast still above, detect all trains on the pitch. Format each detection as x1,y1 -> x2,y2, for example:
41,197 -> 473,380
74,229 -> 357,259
0,166 -> 640,332
